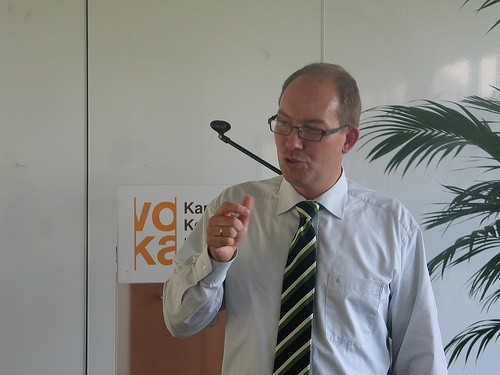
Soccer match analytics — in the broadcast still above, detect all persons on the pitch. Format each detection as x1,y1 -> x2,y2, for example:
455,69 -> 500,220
161,61 -> 449,375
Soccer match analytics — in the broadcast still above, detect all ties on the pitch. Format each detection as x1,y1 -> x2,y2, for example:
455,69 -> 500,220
272,201 -> 322,375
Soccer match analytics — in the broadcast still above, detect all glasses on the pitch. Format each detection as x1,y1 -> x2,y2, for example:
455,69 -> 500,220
268,114 -> 349,142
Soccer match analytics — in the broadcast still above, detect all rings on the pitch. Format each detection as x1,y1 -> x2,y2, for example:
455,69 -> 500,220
219,226 -> 223,237
